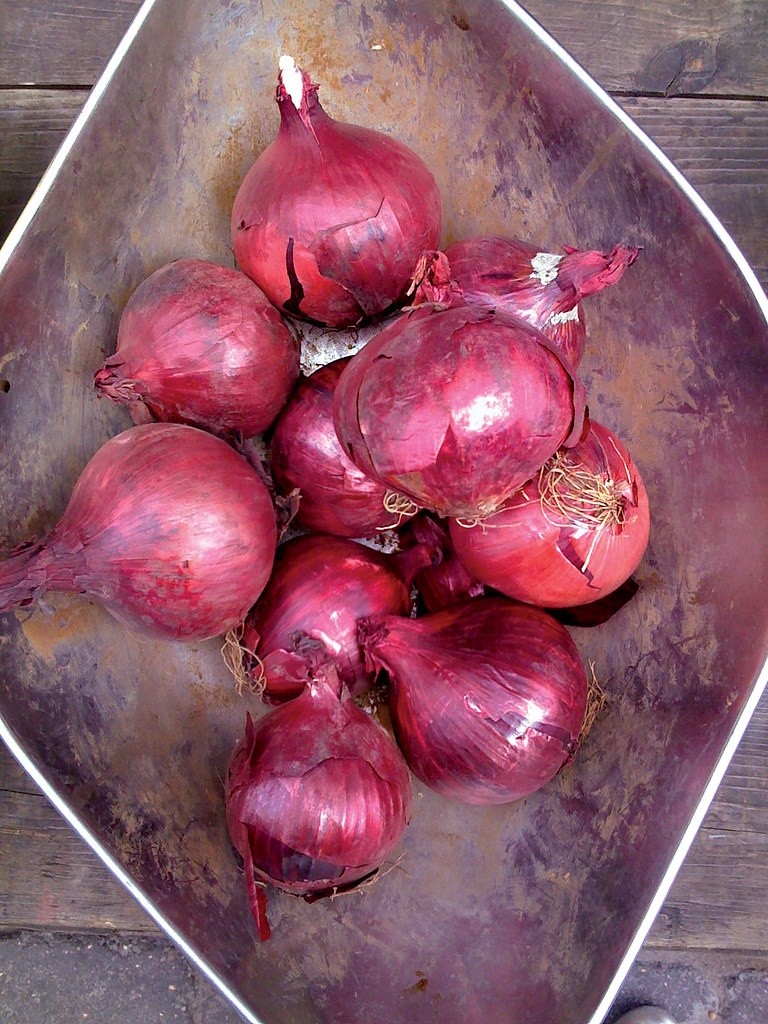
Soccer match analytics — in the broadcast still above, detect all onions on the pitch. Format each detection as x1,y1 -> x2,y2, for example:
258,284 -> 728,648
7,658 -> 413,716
0,54 -> 651,904
